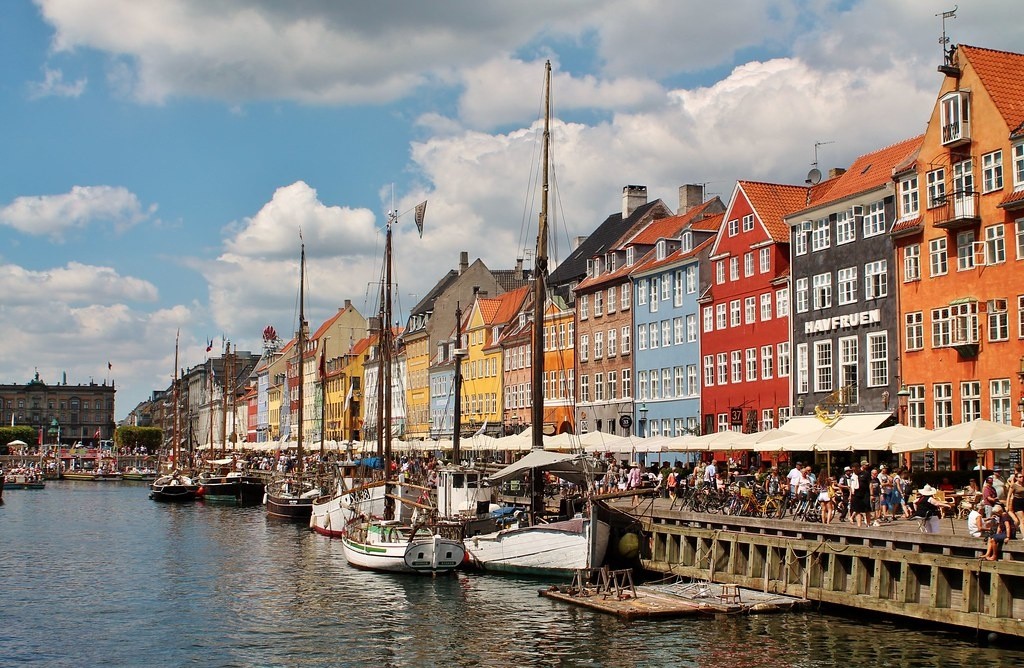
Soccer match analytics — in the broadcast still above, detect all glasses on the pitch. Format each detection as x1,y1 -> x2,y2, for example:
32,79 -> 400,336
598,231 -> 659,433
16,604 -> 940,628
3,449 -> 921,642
1017,476 -> 1023,478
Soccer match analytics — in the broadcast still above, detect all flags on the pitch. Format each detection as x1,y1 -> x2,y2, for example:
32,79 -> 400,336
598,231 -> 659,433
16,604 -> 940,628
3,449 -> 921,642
94,429 -> 100,438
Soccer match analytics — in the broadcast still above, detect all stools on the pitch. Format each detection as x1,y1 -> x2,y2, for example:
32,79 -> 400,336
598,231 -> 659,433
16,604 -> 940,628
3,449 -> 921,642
718,584 -> 742,605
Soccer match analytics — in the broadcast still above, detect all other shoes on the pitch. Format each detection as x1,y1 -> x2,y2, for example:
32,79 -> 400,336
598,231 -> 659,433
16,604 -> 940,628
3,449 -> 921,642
906,514 -> 914,519
979,554 -> 990,558
1016,530 -> 1021,533
892,517 -> 898,521
839,515 -> 882,528
986,555 -> 997,561
884,518 -> 891,523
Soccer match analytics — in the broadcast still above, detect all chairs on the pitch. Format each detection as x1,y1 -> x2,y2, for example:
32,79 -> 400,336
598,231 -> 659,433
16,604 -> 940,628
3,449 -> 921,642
934,489 -> 956,519
958,494 -> 982,520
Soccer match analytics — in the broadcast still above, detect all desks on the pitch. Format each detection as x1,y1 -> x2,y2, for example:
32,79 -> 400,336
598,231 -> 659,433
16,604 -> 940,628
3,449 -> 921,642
941,490 -> 955,497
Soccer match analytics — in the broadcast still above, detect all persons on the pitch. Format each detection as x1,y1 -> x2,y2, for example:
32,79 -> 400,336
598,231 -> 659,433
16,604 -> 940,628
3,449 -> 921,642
0,443 -> 1024,563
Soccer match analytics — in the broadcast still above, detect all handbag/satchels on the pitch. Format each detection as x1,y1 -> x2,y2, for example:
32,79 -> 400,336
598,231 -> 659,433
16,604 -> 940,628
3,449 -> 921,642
689,476 -> 697,487
763,474 -> 773,489
622,476 -> 628,483
827,486 -> 835,498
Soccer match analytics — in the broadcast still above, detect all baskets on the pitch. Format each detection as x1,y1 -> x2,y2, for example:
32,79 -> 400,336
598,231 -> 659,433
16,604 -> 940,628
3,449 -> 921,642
741,487 -> 752,496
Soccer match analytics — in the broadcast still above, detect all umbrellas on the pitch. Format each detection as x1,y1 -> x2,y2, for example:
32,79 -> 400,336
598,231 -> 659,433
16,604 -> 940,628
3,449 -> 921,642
195,418 -> 1024,486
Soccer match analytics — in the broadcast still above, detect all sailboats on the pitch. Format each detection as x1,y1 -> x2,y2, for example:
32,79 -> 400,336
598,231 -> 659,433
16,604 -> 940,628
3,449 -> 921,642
0,59 -> 612,585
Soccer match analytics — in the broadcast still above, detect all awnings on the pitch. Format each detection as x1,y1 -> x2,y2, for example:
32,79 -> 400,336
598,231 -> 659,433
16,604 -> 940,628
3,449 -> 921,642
780,414 -> 892,430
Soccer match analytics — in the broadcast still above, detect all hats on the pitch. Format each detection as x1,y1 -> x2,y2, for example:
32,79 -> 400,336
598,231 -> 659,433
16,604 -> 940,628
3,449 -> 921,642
796,462 -> 803,466
918,484 -> 937,495
733,471 -> 739,474
880,465 -> 888,469
844,466 -> 853,472
993,465 -> 1003,471
630,461 -> 638,466
860,460 -> 869,466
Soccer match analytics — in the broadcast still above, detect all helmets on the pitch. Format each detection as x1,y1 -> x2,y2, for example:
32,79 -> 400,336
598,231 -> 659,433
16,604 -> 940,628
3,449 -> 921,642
986,477 -> 993,483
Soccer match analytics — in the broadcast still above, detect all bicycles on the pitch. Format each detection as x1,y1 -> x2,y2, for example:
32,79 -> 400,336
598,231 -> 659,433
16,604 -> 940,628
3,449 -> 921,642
669,477 -> 846,525
